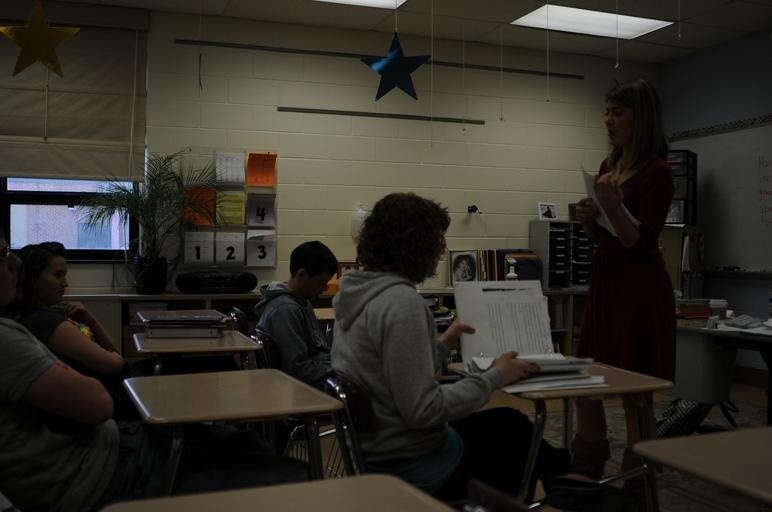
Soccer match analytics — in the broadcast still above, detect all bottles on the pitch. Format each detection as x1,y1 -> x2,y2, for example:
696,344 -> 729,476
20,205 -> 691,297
707,297 -> 727,321
507,265 -> 517,282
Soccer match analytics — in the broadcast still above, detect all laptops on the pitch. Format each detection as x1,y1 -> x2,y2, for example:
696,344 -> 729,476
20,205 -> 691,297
136,309 -> 229,330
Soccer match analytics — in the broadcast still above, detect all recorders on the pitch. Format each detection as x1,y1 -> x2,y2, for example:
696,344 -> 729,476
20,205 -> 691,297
175,267 -> 258,293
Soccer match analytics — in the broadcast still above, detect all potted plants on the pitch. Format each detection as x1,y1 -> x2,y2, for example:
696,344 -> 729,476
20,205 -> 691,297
76,146 -> 227,296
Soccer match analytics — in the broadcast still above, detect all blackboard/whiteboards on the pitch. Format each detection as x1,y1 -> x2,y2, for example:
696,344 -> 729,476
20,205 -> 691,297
668,114 -> 772,280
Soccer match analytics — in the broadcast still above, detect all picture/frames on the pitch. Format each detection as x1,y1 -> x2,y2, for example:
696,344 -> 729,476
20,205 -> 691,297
337,262 -> 359,281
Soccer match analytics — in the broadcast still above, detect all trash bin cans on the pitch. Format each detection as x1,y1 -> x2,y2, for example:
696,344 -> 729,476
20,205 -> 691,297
675,320 -> 736,403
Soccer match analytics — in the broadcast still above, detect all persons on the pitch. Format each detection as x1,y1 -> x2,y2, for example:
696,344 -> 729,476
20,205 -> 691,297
571,76 -> 674,510
453,254 -> 474,282
1,234 -> 310,512
17,241 -> 238,417
255,240 -> 339,400
542,206 -> 554,217
328,192 -> 610,511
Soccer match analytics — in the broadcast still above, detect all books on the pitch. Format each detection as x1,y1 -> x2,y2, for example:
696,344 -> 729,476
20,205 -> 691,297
672,290 -> 772,336
665,150 -> 695,221
452,280 -> 609,395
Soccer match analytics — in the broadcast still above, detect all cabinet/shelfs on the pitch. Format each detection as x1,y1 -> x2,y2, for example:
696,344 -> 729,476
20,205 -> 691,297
662,150 -> 697,227
529,219 -> 594,290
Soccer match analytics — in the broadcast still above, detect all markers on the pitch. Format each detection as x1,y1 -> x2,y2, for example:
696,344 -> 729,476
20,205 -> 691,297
716,266 -> 766,272
